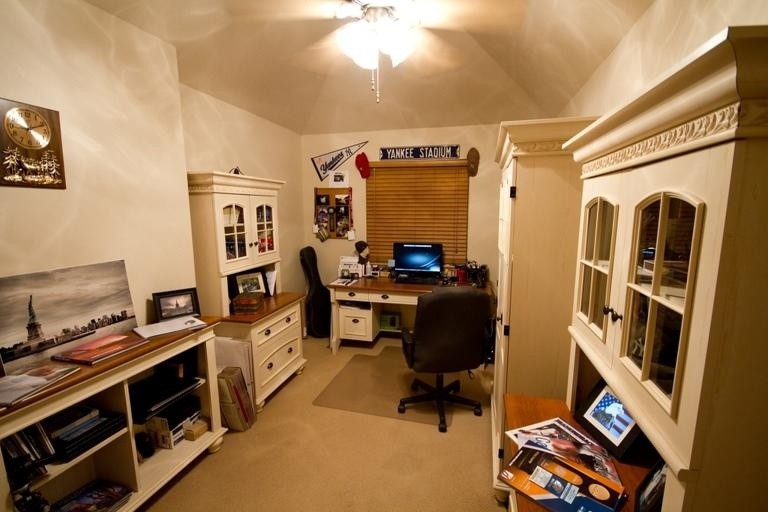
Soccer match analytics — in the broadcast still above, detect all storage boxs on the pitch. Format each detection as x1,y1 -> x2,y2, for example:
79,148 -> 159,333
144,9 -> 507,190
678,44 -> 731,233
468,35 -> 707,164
233,293 -> 264,315
146,394 -> 201,450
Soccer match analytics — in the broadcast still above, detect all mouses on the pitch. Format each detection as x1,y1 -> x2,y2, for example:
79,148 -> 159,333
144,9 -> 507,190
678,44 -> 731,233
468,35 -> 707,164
442,280 -> 448,285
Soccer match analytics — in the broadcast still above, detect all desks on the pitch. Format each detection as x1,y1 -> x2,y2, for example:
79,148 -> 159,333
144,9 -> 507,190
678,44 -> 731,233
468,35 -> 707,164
326,277 -> 494,356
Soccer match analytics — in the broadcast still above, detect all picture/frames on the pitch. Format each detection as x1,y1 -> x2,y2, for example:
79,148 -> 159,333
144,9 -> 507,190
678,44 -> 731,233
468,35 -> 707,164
329,171 -> 349,187
152,287 -> 201,323
572,377 -> 641,461
233,269 -> 271,300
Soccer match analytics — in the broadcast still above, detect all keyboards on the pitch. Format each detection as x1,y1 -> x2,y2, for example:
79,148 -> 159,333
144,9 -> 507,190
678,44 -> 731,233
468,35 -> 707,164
397,276 -> 436,285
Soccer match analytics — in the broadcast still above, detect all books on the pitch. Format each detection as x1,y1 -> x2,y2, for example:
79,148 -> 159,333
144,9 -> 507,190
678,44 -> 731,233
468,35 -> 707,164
44,403 -> 100,439
48,479 -> 135,512
495,416 -> 630,511
2,421 -> 56,469
52,334 -> 150,366
233,293 -> 265,314
0,364 -> 81,407
132,314 -> 207,340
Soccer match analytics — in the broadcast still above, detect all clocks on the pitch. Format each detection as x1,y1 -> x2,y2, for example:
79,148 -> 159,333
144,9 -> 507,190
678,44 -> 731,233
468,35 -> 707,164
0,98 -> 68,191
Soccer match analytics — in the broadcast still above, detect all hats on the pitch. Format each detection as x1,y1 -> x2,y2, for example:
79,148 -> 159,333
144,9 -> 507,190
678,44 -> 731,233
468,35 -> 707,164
356,152 -> 370,178
467,148 -> 479,176
355,241 -> 368,253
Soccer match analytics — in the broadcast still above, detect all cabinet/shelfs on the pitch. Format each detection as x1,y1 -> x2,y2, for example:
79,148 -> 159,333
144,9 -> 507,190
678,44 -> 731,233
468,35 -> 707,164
510,25 -> 768,510
1,316 -> 229,512
187,171 -> 309,414
489,115 -> 595,491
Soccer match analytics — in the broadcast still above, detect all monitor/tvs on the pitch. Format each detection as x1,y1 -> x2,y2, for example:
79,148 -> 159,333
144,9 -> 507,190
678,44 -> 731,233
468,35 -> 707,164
393,242 -> 443,277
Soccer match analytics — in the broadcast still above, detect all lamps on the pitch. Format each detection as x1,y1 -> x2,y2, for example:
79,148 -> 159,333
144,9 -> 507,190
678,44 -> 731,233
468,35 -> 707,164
331,1 -> 419,107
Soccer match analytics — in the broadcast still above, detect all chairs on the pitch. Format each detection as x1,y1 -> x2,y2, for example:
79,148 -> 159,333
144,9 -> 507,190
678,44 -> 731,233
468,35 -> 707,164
398,287 -> 492,434
